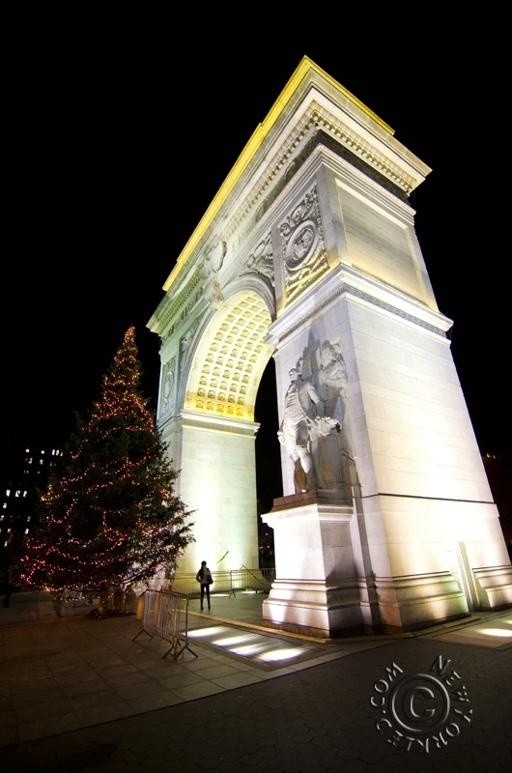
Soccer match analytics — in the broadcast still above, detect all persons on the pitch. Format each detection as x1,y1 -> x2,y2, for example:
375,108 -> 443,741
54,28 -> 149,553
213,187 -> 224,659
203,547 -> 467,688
196,561 -> 213,610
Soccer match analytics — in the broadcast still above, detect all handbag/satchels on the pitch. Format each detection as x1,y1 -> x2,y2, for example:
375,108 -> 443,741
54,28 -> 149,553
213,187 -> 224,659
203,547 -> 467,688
206,575 -> 212,584
